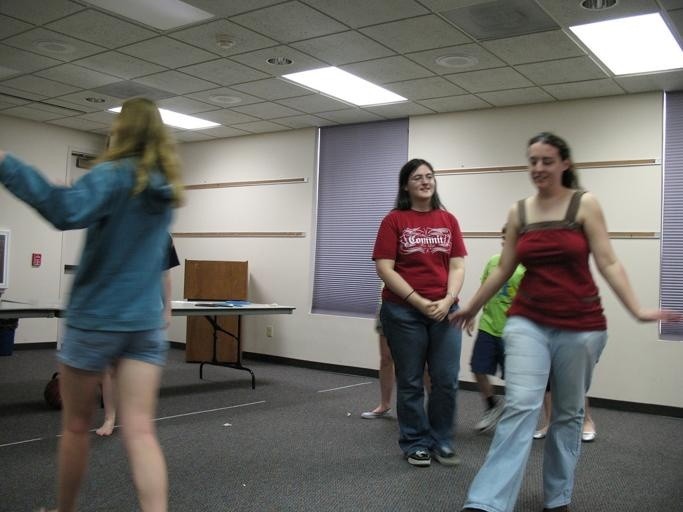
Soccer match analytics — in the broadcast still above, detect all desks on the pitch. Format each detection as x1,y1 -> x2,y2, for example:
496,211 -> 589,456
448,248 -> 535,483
0,300 -> 59,322
1,297 -> 298,413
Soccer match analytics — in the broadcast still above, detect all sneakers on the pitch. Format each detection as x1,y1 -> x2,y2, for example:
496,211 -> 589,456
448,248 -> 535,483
531,419 -> 555,439
405,444 -> 433,465
580,417 -> 597,441
431,445 -> 462,466
361,405 -> 398,419
474,397 -> 509,434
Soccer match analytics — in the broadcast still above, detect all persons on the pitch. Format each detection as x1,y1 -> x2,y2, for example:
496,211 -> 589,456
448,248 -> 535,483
462,219 -> 528,436
357,291 -> 463,442
369,157 -> 468,470
92,228 -> 180,440
0,95 -> 189,512
530,365 -> 599,444
446,130 -> 682,512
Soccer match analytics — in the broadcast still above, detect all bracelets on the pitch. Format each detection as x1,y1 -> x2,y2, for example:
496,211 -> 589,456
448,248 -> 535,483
402,288 -> 416,303
